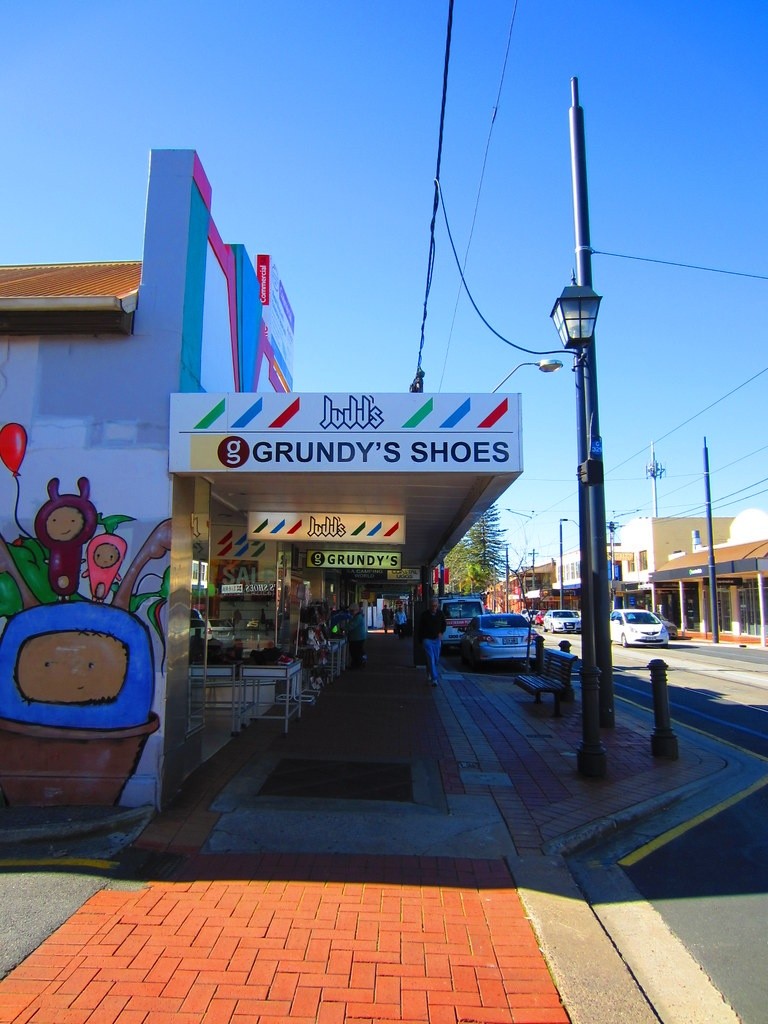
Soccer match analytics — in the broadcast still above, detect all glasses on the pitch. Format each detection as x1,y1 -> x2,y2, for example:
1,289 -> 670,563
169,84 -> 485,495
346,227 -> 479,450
350,610 -> 356,612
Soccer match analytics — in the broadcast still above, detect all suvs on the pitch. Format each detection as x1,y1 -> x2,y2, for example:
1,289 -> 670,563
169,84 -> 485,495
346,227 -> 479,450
205,619 -> 235,645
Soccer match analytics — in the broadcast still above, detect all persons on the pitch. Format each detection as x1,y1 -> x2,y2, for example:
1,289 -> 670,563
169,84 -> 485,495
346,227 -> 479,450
382,605 -> 390,633
390,609 -> 394,624
419,598 -> 446,687
395,606 -> 407,634
345,604 -> 368,667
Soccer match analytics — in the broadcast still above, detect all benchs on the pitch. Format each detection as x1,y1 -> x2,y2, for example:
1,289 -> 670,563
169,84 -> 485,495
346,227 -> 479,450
514,648 -> 578,717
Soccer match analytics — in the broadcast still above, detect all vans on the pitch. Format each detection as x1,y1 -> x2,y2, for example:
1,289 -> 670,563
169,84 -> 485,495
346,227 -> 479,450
441,598 -> 485,654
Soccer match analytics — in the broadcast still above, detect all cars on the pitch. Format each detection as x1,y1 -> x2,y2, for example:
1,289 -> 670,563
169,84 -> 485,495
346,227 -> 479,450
535,610 -> 547,626
458,613 -> 539,669
544,609 -> 581,633
652,612 -> 678,640
610,608 -> 669,647
521,610 -> 538,622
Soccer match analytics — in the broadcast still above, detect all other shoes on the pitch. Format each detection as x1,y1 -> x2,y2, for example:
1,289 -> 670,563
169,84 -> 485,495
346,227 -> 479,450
432,680 -> 438,688
426,672 -> 431,680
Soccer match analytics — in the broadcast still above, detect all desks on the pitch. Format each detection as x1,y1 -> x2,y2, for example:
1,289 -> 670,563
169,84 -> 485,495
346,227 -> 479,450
236,658 -> 303,737
188,662 -> 250,737
302,637 -> 348,683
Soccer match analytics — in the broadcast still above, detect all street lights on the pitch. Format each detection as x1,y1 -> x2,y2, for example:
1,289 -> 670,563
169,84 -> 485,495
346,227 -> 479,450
549,269 -> 609,779
